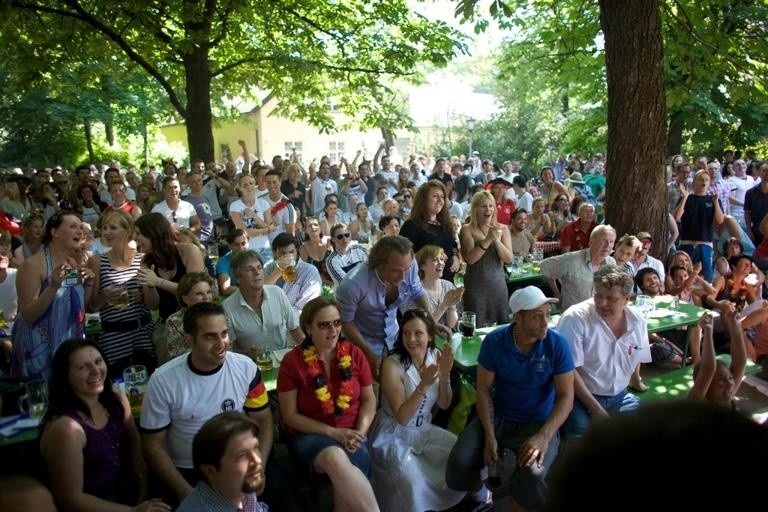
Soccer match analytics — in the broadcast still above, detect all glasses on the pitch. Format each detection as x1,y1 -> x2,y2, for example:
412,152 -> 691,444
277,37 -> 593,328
337,232 -> 350,239
317,320 -> 342,329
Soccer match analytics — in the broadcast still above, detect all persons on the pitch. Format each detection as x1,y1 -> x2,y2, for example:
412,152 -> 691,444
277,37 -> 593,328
1,140 -> 767,511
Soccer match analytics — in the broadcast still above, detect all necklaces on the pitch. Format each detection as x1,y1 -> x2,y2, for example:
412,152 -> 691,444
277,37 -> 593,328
300,338 -> 352,415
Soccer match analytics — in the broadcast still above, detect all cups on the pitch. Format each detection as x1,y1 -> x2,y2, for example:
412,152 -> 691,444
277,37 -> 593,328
635,294 -> 656,319
0,304 -> 5,330
254,343 -> 272,372
208,243 -> 219,262
532,248 -> 544,273
106,280 -> 130,309
457,311 -> 477,340
19,379 -> 49,418
123,364 -> 149,408
511,256 -> 524,278
276,253 -> 299,282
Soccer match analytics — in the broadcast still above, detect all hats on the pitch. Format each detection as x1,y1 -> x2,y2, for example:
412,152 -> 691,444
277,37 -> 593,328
485,178 -> 513,189
637,231 -> 654,243
566,171 -> 585,184
508,285 -> 560,314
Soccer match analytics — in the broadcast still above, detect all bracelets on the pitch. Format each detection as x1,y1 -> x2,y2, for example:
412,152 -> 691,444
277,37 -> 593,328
478,244 -> 488,251
629,377 -> 642,385
416,386 -> 425,396
439,380 -> 450,385
83,282 -> 94,287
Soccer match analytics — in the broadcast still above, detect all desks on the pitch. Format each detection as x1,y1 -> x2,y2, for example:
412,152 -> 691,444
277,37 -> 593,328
432,295 -> 718,386
1,356 -> 280,456
456,259 -> 560,298
533,241 -> 561,254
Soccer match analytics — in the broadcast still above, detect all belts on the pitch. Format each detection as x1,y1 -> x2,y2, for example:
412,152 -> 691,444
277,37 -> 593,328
102,313 -> 151,333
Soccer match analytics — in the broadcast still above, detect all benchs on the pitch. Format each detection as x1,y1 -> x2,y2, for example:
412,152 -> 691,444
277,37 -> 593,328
631,350 -> 761,406
